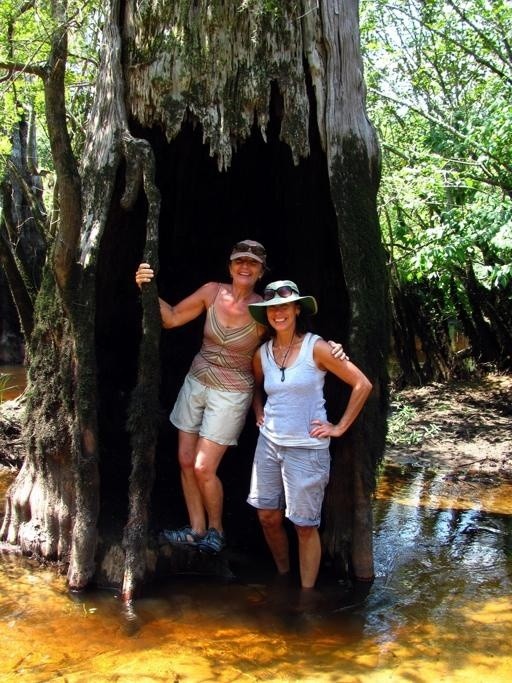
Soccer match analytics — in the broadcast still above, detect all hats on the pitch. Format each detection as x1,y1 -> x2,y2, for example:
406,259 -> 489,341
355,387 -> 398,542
230,240 -> 267,267
247,280 -> 318,328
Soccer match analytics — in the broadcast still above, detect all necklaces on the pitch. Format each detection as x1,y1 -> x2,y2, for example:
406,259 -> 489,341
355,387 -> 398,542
265,330 -> 295,382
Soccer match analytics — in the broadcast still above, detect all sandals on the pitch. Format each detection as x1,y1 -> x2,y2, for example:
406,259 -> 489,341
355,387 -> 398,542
165,527 -> 204,546
200,527 -> 226,552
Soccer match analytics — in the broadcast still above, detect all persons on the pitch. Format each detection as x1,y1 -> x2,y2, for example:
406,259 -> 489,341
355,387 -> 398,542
245,280 -> 373,613
134,237 -> 351,559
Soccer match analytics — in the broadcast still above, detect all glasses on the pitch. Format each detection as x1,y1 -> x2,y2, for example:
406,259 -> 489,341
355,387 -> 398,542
262,286 -> 300,301
234,242 -> 267,255
